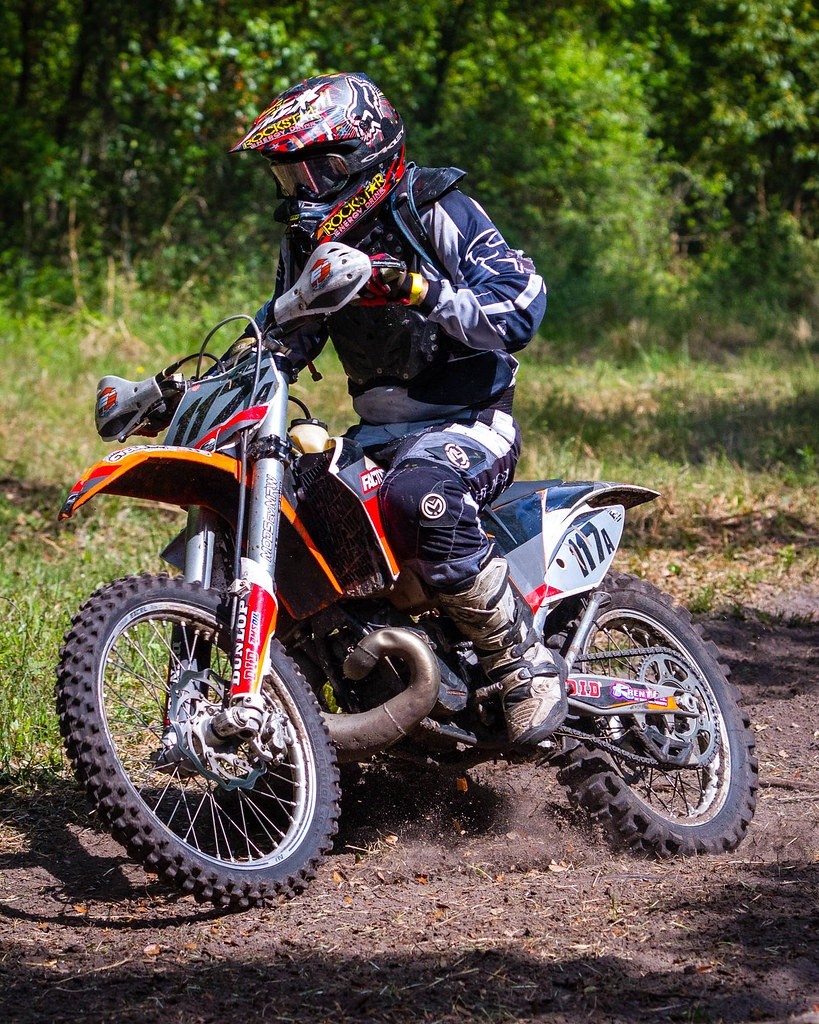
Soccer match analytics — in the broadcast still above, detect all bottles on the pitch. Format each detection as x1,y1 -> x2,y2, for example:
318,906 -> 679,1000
289,417 -> 330,453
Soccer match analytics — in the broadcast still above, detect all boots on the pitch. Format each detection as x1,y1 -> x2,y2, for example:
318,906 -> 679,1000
439,554 -> 569,750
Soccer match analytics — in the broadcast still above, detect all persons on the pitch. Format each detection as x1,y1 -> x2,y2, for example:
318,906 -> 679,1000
101,69 -> 575,755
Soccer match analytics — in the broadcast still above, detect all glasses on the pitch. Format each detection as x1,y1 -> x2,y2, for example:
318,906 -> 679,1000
265,152 -> 351,199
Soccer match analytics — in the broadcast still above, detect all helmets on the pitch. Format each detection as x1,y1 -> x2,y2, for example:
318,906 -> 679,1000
231,74 -> 406,256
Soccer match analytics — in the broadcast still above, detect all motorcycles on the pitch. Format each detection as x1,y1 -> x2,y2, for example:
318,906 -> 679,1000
55,240 -> 759,910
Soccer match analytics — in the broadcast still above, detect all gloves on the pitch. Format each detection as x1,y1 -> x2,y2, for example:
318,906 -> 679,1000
352,248 -> 411,313
